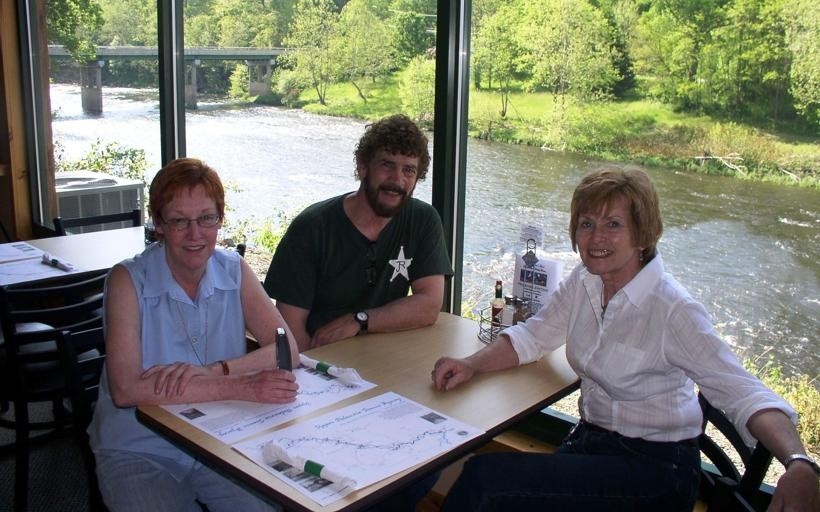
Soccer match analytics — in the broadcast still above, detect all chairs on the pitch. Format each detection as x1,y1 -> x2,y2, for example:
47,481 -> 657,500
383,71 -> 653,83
55,327 -> 108,511
53,210 -> 141,236
0,274 -> 108,511
693,386 -> 777,512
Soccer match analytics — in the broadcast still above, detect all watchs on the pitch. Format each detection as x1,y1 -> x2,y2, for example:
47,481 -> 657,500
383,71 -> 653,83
352,310 -> 369,336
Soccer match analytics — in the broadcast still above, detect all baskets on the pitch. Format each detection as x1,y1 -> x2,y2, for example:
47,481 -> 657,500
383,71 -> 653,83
478,239 -> 537,346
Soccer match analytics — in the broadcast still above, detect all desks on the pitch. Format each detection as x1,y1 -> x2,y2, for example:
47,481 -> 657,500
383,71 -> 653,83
0,226 -> 158,290
135,311 -> 580,511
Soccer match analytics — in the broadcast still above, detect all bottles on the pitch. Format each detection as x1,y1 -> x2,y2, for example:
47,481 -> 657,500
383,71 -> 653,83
492,280 -> 531,327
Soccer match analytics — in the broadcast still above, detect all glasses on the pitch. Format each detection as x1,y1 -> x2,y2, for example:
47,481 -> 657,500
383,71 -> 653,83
156,210 -> 223,231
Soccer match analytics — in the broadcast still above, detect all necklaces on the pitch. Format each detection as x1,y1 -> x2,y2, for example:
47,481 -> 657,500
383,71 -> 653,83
600,279 -> 611,319
173,300 -> 209,367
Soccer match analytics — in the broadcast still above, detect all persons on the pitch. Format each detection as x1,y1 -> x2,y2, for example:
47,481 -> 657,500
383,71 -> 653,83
86,158 -> 299,512
263,114 -> 454,353
431,165 -> 820,512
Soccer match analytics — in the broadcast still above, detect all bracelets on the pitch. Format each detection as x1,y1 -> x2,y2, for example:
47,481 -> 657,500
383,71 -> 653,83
784,453 -> 820,475
214,361 -> 229,375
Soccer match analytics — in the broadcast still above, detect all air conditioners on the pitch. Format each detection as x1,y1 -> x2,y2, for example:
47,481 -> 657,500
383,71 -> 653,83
54,170 -> 138,235
